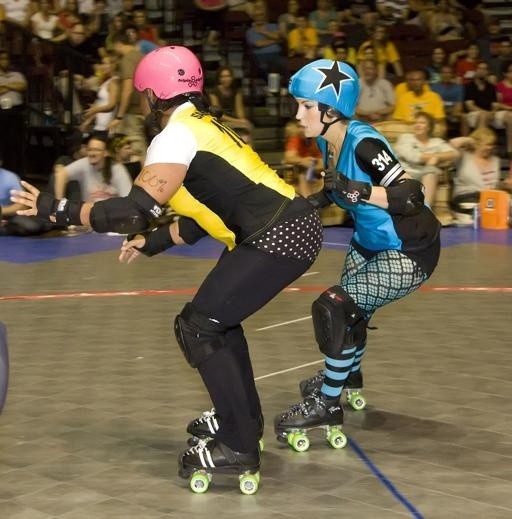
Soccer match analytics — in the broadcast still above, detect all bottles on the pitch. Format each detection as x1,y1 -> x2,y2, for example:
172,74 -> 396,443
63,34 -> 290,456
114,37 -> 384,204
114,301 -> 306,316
473,206 -> 479,230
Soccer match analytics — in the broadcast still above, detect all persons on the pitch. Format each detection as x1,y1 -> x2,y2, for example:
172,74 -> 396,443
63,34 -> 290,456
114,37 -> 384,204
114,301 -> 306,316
9,44 -> 322,472
1,1 -> 510,233
272,59 -> 441,432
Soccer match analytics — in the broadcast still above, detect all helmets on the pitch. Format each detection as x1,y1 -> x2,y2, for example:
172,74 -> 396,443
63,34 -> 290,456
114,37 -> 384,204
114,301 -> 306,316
289,59 -> 361,119
134,46 -> 203,100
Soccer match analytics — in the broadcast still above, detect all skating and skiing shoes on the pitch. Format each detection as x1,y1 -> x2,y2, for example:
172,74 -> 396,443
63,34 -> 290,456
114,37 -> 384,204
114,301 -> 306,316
299,369 -> 367,411
178,408 -> 264,495
274,387 -> 347,452
